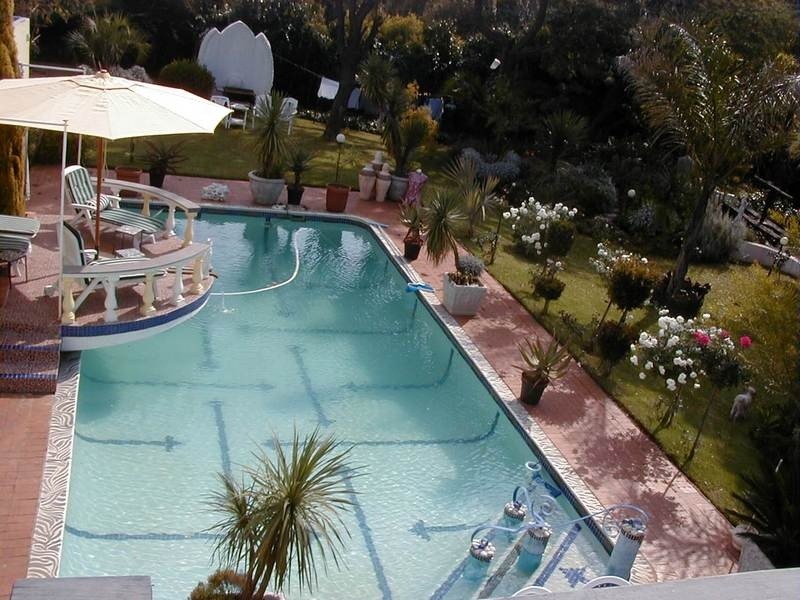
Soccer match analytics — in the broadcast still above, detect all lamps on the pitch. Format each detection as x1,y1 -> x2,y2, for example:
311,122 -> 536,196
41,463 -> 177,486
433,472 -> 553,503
780,237 -> 788,245
627,189 -> 635,197
336,133 -> 346,144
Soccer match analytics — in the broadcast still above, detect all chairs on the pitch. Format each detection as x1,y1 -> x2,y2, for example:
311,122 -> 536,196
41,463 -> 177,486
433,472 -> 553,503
44,165 -> 165,299
209,96 -> 231,129
0,214 -> 41,254
250,95 -> 299,135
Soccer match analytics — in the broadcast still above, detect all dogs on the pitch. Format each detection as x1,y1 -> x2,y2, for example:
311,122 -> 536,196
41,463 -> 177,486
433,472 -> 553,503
729,386 -> 756,423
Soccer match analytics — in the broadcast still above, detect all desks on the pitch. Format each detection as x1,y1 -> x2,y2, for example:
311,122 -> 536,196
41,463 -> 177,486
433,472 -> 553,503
113,225 -> 143,250
115,248 -> 145,259
227,104 -> 249,130
0,247 -> 28,289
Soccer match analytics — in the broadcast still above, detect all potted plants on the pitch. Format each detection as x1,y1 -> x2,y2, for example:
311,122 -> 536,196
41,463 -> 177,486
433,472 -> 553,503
325,134 -> 360,213
511,327 -> 575,407
399,154 -> 502,317
116,137 -> 191,197
285,146 -> 322,204
186,419 -> 371,600
247,90 -> 293,206
